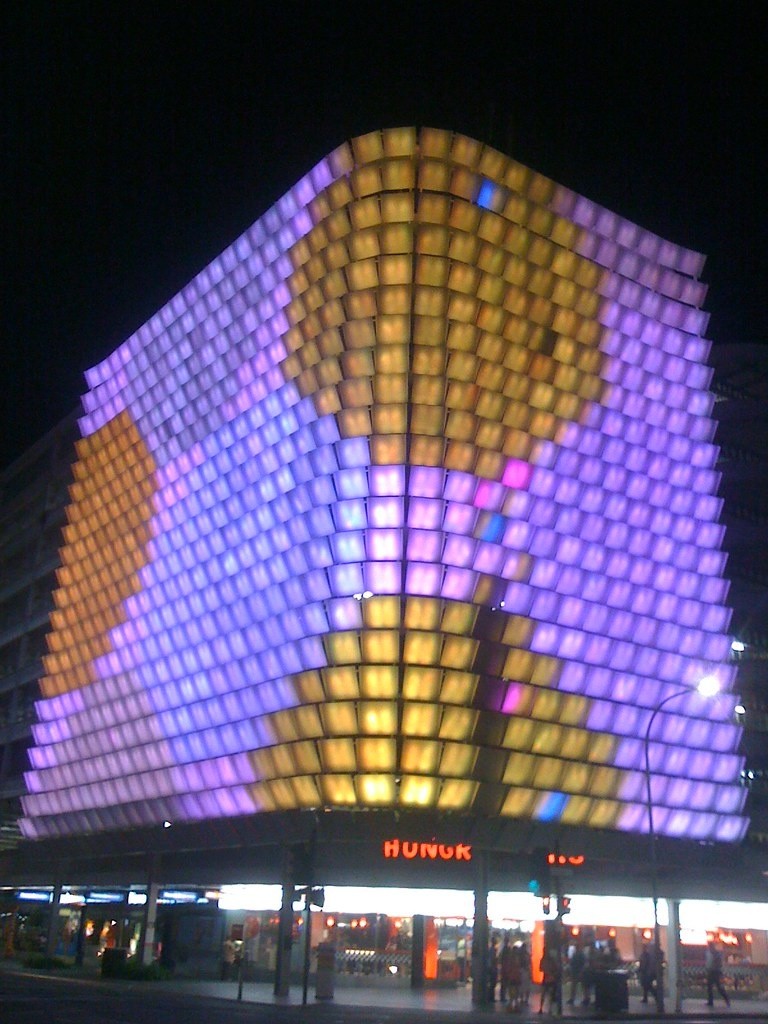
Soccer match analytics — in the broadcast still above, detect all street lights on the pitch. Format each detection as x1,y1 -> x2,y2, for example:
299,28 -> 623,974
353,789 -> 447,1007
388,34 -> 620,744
646,675 -> 720,1014
137,822 -> 171,963
302,806 -> 333,1004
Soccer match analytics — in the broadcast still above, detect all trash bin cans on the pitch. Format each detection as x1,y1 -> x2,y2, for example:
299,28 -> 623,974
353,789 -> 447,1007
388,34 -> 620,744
101,947 -> 127,978
594,968 -> 630,1012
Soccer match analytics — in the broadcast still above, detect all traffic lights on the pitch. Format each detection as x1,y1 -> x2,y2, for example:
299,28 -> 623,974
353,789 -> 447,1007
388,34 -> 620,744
543,896 -> 571,915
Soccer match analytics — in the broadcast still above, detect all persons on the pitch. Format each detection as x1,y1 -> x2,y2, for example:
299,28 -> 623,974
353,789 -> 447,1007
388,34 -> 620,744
539,948 -> 563,1016
487,931 -> 531,1014
705,941 -> 732,1008
567,939 -> 621,1006
220,936 -> 235,981
639,941 -> 657,1003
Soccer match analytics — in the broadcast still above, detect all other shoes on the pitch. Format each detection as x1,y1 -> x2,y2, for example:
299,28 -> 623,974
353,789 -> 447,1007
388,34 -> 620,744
705,1002 -> 714,1006
640,999 -> 647,1003
